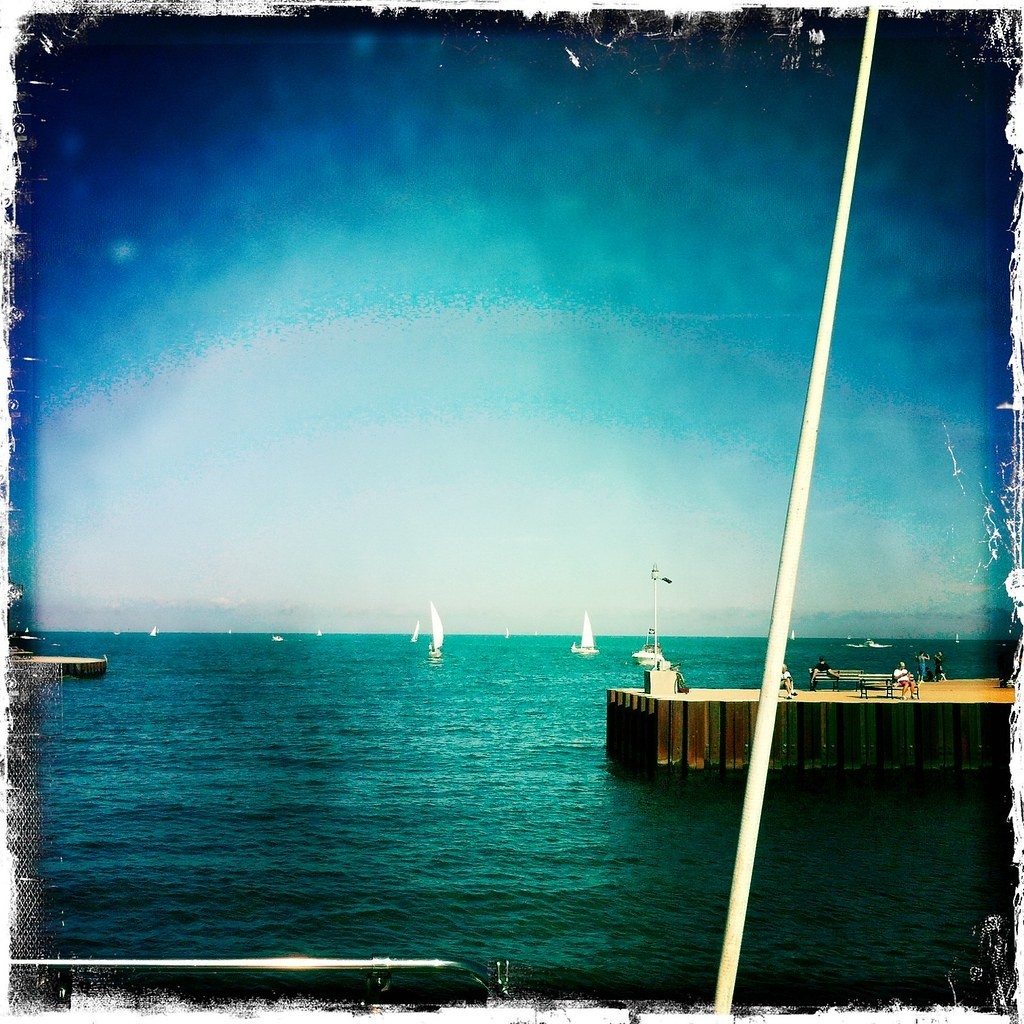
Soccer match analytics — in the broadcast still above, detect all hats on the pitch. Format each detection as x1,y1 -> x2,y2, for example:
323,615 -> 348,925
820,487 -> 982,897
899,662 -> 905,667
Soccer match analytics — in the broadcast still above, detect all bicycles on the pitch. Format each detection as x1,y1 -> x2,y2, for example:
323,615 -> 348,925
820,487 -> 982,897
670,662 -> 686,692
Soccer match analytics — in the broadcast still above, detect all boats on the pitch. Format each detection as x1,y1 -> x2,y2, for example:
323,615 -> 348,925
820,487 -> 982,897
632,627 -> 665,667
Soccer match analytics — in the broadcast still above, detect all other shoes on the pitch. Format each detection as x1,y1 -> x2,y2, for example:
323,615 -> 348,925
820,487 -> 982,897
786,696 -> 792,699
791,692 -> 798,696
921,680 -> 924,684
911,695 -> 915,699
904,697 -> 907,700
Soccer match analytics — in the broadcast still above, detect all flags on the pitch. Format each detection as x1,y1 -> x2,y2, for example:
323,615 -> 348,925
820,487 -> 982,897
648,628 -> 655,635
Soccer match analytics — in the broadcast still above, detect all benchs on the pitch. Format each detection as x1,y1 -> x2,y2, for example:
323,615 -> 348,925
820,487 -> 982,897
808,668 -> 866,692
856,674 -> 920,700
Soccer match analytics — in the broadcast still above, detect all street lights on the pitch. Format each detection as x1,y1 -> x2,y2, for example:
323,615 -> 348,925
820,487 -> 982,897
651,563 -> 672,672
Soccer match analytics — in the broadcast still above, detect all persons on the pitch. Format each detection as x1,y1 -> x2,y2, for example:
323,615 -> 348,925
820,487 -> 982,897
894,662 -> 918,700
915,650 -> 947,683
999,645 -> 1022,688
810,656 -> 840,692
780,664 -> 798,699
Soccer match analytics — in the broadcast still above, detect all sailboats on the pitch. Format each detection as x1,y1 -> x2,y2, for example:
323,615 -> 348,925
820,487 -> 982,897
411,619 -> 420,643
571,611 -> 600,654
428,600 -> 444,658
